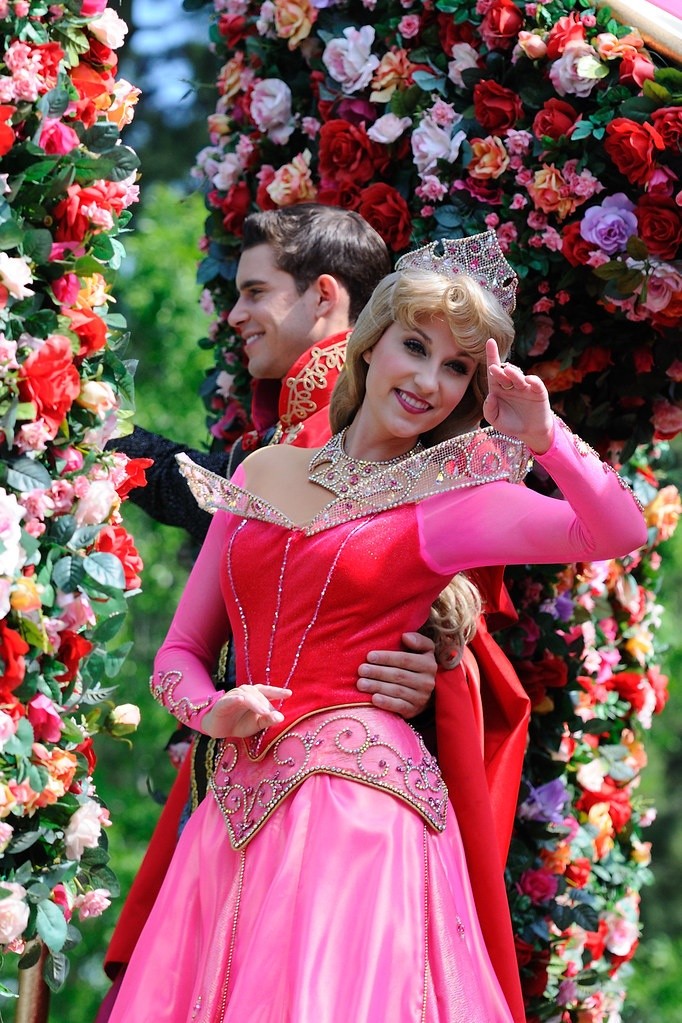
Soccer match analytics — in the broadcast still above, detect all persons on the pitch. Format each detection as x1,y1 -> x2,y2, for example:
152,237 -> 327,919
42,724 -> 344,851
91,206 -> 533,1023
106,230 -> 648,1023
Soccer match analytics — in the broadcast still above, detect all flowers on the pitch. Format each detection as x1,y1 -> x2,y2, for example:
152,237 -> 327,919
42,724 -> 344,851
199,2 -> 682,1023
0,2 -> 150,991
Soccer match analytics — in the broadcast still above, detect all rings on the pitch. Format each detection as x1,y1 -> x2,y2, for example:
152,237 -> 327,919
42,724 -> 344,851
501,384 -> 515,392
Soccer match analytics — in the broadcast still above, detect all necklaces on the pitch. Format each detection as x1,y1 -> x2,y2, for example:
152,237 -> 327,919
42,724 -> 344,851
306,420 -> 434,509
